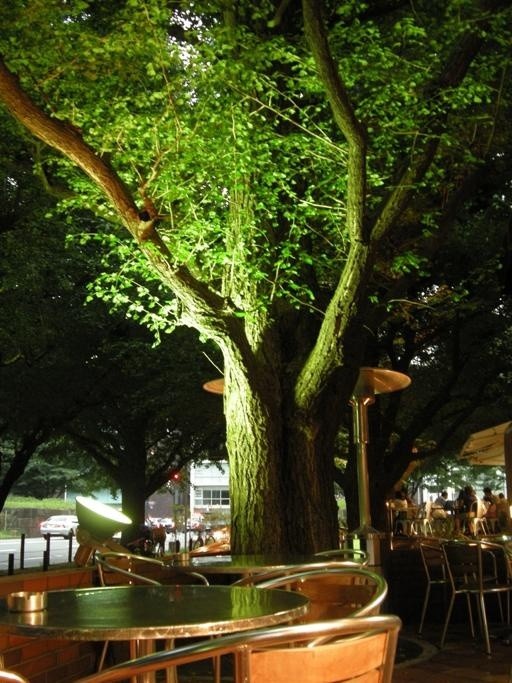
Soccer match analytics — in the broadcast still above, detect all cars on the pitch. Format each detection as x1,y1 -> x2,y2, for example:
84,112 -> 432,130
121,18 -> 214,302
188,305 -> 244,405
151,517 -> 177,534
39,515 -> 80,540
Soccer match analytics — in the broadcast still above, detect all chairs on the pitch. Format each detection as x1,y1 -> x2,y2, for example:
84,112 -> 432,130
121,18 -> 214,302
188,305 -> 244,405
0,535 -> 403,682
378,496 -> 511,657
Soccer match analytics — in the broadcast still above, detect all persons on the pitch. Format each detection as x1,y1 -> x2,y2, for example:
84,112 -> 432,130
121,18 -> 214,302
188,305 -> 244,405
400,486 -> 416,520
205,519 -> 212,535
434,485 -> 511,537
391,490 -> 408,537
